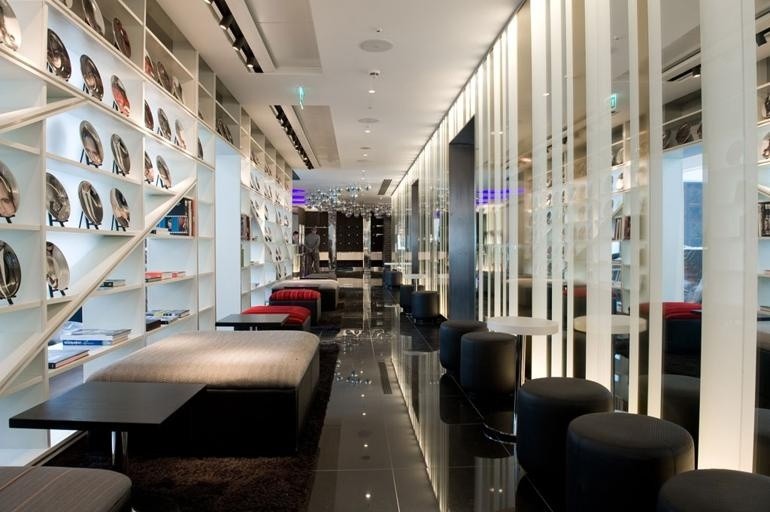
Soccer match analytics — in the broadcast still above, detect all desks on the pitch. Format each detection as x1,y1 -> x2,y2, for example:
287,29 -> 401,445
403,272 -> 428,292
384,262 -> 399,271
215,314 -> 289,330
9,381 -> 208,473
481,316 -> 564,449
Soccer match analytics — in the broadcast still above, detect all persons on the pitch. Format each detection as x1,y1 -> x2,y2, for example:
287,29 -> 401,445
292,230 -> 300,243
306,227 -> 322,275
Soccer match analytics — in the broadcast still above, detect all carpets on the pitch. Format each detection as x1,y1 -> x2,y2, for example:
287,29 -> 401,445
42,342 -> 338,511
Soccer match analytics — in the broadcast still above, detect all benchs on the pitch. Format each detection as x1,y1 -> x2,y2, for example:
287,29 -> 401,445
513,277 -> 701,428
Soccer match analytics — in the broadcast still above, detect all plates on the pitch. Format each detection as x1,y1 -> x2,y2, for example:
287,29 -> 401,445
756,88 -> 769,162
527,146 -> 624,280
2,1 -> 23,53
46,1 -> 292,289
2,162 -> 20,219
664,128 -> 672,149
696,120 -> 702,139
1,241 -> 24,300
674,123 -> 692,144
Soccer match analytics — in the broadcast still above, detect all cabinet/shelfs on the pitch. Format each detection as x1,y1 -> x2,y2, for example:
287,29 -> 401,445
0,0 -> 223,469
216,74 -> 239,152
488,61 -> 770,344
216,105 -> 300,320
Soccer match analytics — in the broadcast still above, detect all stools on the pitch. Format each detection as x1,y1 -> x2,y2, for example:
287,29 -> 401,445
438,317 -> 488,376
400,284 -> 423,312
1,466 -> 132,512
514,376 -> 614,480
565,409 -> 694,511
380,268 -> 388,283
85,331 -> 320,433
387,270 -> 401,289
655,467 -> 769,512
459,331 -> 518,397
410,291 -> 440,325
241,267 -> 338,330
